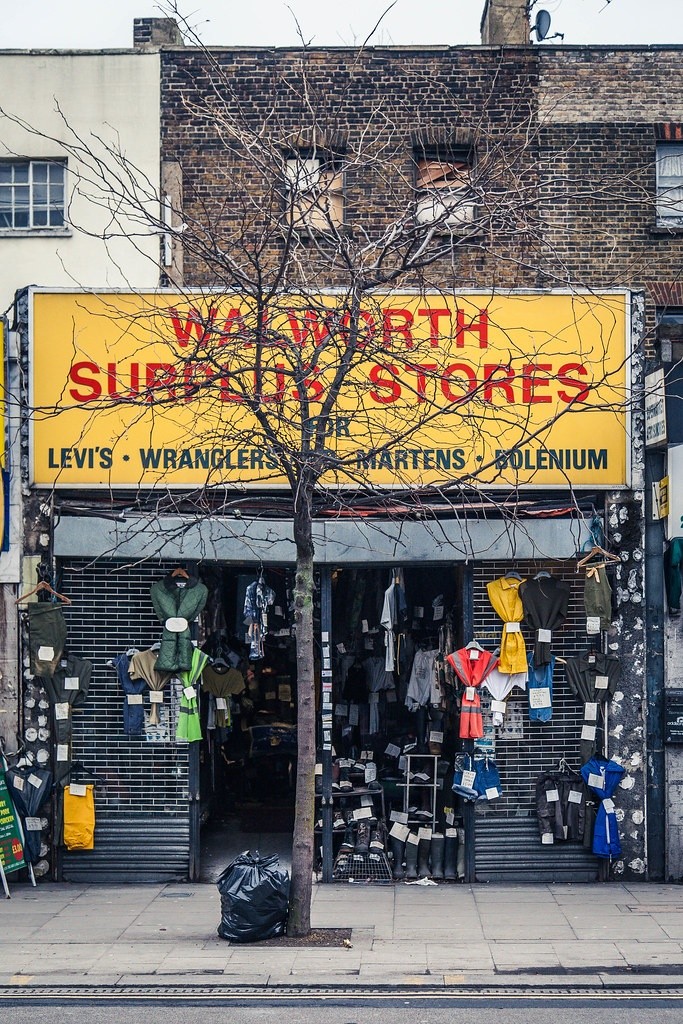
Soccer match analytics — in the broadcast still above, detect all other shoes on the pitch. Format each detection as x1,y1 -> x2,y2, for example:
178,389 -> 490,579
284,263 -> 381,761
314,706 -> 446,791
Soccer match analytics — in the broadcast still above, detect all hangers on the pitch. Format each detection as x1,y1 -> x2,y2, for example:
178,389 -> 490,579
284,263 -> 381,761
170,559 -> 189,580
465,740 -> 488,761
14,562 -> 72,606
443,536 -> 622,661
541,758 -> 584,782
53,756 -> 108,789
256,562 -> 404,587
591,741 -> 610,763
14,744 -> 34,769
60,633 -> 232,670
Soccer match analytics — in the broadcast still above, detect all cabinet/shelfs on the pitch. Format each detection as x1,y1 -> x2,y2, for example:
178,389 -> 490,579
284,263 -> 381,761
395,751 -> 444,835
313,772 -> 388,872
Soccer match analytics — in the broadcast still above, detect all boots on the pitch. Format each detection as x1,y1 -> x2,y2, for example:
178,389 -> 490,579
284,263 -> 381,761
405,841 -> 417,877
390,835 -> 405,878
445,836 -> 457,880
419,838 -> 431,879
431,837 -> 445,879
457,840 -> 466,878
409,787 -> 433,821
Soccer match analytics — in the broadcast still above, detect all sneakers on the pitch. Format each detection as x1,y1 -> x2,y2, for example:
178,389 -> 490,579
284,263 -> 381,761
317,805 -> 385,855
